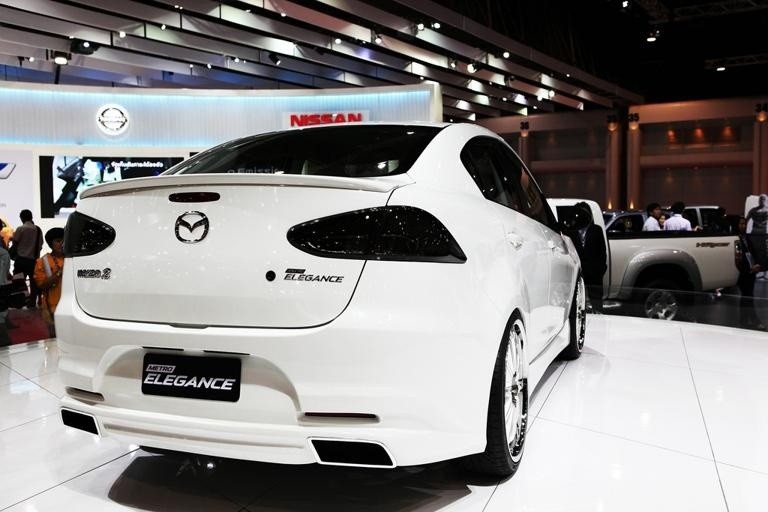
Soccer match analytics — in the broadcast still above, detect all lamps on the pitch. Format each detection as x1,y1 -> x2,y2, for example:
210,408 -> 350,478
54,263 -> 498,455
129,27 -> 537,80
71,39 -> 93,54
54,51 -> 68,65
269,52 -> 281,65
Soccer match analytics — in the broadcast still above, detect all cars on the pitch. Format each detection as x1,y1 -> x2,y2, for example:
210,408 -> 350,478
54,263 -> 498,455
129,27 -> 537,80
546,197 -> 743,321
52,121 -> 589,487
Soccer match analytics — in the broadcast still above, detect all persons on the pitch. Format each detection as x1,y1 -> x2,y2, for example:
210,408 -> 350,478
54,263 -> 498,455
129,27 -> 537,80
745,194 -> 768,281
0,208 -> 64,349
642,201 -> 767,331
566,201 -> 608,315
